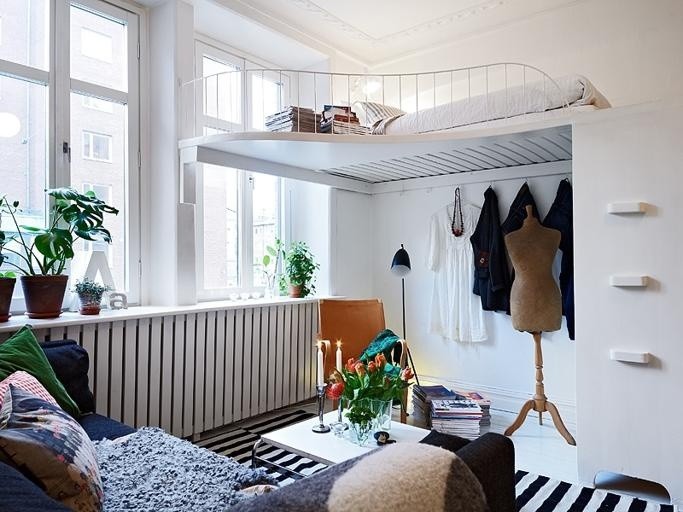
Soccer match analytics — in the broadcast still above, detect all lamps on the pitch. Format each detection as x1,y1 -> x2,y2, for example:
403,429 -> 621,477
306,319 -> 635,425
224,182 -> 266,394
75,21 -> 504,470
390,242 -> 422,390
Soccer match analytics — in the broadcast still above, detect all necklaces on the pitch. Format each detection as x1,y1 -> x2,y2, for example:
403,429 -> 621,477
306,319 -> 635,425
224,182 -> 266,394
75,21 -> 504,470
451,187 -> 464,237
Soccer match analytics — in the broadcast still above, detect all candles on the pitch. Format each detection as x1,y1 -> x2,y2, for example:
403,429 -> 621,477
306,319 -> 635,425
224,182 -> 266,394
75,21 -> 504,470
314,337 -> 325,385
334,338 -> 344,374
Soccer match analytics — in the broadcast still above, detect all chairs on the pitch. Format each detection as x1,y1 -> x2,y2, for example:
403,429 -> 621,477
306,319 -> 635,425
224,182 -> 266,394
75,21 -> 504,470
317,298 -> 408,422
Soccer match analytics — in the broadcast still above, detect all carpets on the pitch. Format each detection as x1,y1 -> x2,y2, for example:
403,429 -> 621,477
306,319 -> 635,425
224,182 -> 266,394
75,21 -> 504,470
183,407 -> 680,512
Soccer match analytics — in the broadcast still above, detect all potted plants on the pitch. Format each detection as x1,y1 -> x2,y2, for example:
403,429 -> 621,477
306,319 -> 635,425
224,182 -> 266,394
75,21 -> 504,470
0,184 -> 119,321
0,231 -> 19,326
71,275 -> 108,316
272,241 -> 318,300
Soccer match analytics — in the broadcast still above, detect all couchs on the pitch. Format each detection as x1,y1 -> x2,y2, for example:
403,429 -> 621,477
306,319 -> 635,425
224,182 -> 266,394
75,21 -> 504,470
0,320 -> 517,512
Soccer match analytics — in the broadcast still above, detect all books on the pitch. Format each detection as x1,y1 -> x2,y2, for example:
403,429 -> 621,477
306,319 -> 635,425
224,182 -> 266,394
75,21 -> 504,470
410,384 -> 493,441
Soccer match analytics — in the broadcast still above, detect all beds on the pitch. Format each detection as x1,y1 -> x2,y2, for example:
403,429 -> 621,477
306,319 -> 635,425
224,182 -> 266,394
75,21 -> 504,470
353,73 -> 613,136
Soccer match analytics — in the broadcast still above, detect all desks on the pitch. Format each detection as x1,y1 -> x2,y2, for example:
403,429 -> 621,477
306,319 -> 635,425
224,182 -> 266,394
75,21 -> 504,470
247,404 -> 433,483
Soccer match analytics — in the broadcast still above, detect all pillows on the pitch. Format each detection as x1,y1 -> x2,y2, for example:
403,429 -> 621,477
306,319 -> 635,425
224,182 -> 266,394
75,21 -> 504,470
0,318 -> 79,412
0,383 -> 103,511
1,364 -> 60,414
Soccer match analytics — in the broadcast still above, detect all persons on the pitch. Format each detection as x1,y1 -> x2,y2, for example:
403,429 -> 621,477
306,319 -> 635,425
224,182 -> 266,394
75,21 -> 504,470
502,217 -> 563,333
